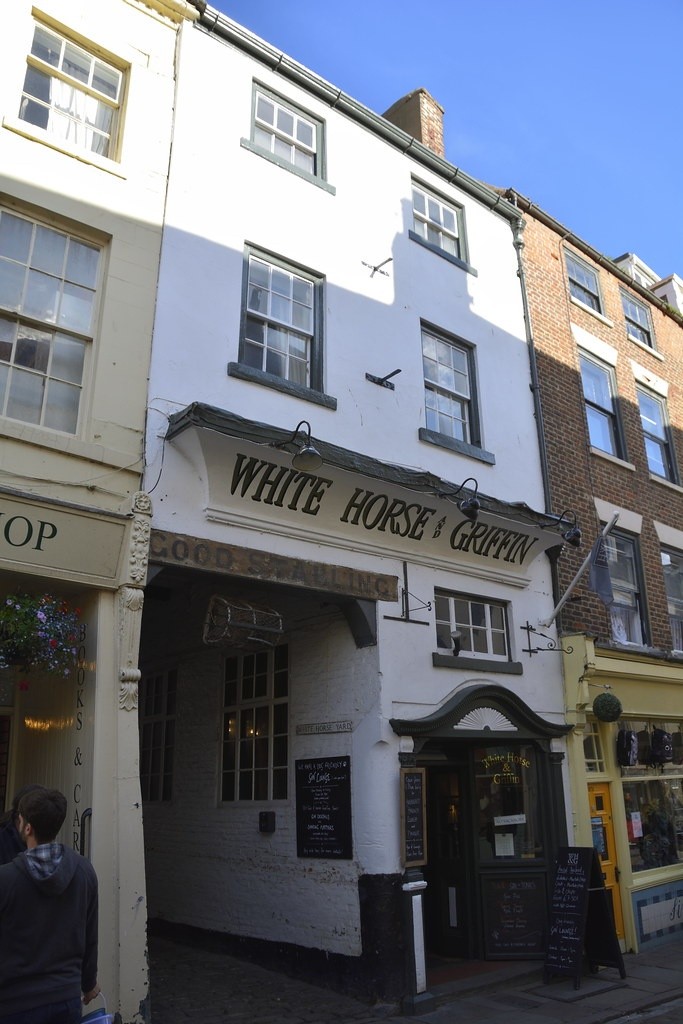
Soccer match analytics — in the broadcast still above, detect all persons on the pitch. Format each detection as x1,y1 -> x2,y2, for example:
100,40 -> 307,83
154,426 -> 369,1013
0,788 -> 101,1024
0,785 -> 45,865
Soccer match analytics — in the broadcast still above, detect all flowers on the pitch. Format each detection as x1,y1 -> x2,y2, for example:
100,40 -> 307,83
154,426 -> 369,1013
0,589 -> 84,681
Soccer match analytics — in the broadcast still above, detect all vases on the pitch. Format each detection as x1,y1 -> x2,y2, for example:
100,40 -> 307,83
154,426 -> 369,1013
6,654 -> 28,665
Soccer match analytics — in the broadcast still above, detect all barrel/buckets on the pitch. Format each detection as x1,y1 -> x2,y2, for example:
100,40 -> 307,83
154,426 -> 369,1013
81,993 -> 114,1024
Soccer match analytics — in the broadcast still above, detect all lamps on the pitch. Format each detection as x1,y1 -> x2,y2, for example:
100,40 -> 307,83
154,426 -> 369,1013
272,421 -> 324,471
434,477 -> 480,521
538,509 -> 581,548
